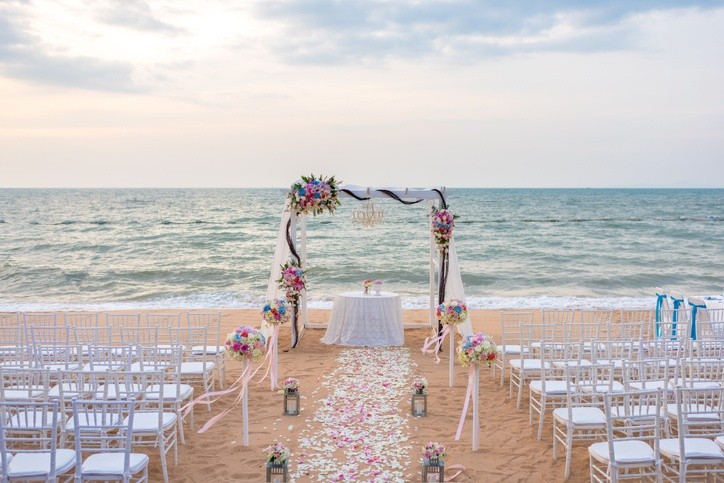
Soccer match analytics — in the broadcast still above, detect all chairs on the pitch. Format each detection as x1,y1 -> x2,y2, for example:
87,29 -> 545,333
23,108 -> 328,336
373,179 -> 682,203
492,287 -> 724,483
0,310 -> 230,483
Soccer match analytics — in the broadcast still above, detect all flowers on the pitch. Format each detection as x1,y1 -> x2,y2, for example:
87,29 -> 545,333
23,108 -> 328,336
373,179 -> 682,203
458,334 -> 499,369
364,280 -> 372,288
222,326 -> 269,362
261,299 -> 292,326
374,280 -> 383,284
283,174 -> 348,217
285,378 -> 299,390
412,376 -> 428,389
263,441 -> 291,466
436,298 -> 471,326
424,204 -> 460,256
273,259 -> 309,319
422,441 -> 447,460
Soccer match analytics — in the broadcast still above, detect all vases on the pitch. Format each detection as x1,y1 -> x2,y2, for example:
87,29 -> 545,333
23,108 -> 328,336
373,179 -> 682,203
273,456 -> 286,465
415,387 -> 423,394
288,389 -> 297,394
374,284 -> 384,296
428,455 -> 439,466
364,286 -> 369,294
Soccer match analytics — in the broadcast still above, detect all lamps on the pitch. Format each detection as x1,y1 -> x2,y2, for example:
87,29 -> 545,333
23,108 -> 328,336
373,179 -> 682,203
353,200 -> 385,227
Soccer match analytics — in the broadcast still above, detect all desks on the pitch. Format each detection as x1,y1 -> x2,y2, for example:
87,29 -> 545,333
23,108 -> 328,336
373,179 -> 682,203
319,291 -> 405,346
422,458 -> 444,483
683,294 -> 724,329
265,462 -> 288,483
412,392 -> 427,416
284,391 -> 300,416
